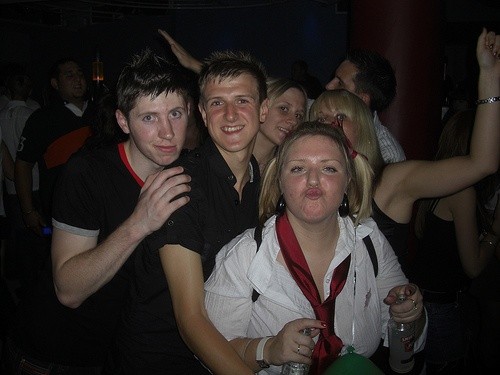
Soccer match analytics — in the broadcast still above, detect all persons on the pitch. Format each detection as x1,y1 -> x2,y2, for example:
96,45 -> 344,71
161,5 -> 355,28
0,27 -> 500,375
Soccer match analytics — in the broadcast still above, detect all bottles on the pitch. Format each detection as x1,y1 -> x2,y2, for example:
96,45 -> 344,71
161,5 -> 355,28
282,327 -> 315,375
388,293 -> 415,373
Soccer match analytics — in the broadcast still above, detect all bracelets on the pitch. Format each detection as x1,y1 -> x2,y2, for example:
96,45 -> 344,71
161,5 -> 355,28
256,336 -> 272,369
240,337 -> 258,362
476,96 -> 500,105
481,240 -> 496,248
22,208 -> 35,216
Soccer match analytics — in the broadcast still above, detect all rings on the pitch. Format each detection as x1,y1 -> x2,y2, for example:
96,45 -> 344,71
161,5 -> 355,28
409,298 -> 416,307
413,306 -> 418,312
297,344 -> 300,352
